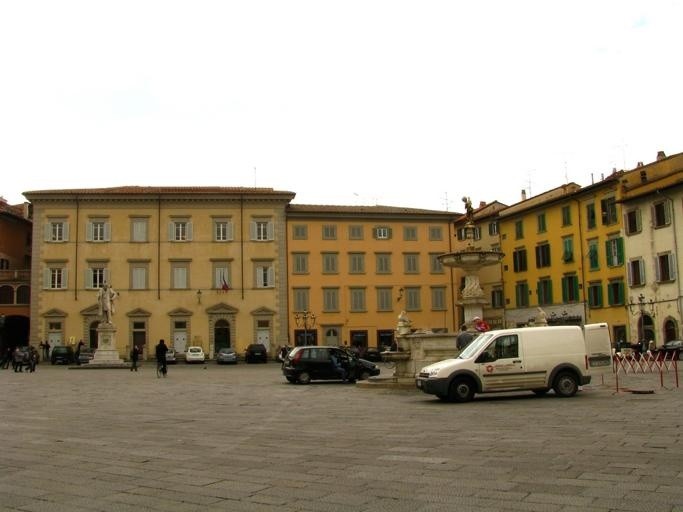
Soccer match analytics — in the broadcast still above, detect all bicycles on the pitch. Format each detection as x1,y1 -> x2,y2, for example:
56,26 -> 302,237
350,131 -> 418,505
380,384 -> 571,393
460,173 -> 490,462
156,362 -> 168,379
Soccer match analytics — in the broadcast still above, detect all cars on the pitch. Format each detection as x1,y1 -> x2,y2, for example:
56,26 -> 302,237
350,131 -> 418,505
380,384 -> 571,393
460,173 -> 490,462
184,346 -> 205,364
165,348 -> 177,361
217,349 -> 237,362
52,345 -> 95,365
277,346 -> 381,385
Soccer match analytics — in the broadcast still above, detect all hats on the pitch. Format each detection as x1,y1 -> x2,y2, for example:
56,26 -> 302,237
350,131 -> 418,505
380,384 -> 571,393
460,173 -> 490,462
471,317 -> 480,322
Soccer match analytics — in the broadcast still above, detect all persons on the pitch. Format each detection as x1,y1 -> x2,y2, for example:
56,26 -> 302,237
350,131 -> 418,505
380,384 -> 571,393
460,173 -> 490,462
96,282 -> 116,325
462,195 -> 474,225
129,344 -> 139,372
473,316 -> 489,332
279,345 -> 288,369
155,339 -> 168,376
0,337 -> 84,373
455,324 -> 473,351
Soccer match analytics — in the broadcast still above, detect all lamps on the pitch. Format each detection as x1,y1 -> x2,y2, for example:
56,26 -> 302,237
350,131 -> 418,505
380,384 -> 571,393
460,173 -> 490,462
197,289 -> 202,304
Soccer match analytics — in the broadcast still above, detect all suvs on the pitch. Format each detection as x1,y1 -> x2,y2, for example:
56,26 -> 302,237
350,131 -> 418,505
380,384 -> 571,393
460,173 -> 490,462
245,344 -> 268,364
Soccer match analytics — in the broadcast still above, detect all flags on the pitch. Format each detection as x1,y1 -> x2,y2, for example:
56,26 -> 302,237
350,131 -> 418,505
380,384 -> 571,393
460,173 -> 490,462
222,278 -> 229,291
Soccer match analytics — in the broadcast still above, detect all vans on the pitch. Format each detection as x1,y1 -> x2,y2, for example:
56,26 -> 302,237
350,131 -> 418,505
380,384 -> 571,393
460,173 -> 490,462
414,323 -> 614,403
